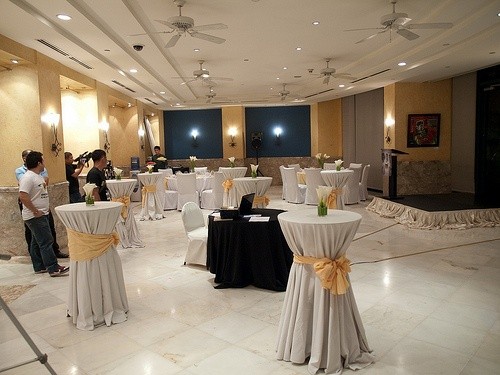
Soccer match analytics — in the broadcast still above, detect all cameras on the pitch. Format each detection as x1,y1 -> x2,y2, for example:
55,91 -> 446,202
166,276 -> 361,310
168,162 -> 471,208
74,151 -> 92,164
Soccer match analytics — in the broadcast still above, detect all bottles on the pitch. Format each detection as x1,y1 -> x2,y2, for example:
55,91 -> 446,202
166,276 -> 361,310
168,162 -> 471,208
131,156 -> 140,170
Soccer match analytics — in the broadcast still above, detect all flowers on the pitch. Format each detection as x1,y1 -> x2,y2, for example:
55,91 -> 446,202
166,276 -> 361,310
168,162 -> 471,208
83,153 -> 344,216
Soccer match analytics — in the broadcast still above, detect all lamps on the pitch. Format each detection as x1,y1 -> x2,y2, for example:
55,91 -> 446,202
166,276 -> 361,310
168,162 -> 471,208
41,108 -> 395,157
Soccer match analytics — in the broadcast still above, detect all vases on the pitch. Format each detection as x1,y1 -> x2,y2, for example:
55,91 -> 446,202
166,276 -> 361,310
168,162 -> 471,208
318,205 -> 328,216
251,172 -> 257,179
85,196 -> 95,206
115,174 -> 122,181
336,166 -> 341,171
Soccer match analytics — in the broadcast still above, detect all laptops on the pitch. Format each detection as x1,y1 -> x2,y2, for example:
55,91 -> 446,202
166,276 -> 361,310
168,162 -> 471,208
227,192 -> 255,215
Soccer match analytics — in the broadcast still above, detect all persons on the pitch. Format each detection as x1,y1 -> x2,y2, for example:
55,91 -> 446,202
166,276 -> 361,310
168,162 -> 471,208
85,149 -> 110,201
15,150 -> 69,257
19,151 -> 69,276
64,152 -> 86,203
152,146 -> 168,172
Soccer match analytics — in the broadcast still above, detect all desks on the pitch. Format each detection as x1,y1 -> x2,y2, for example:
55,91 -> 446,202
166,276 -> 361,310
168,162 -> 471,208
167,166 -> 376,375
54,169 -> 165,331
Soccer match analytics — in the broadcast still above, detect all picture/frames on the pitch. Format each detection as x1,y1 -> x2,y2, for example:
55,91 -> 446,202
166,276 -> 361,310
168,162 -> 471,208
407,113 -> 442,148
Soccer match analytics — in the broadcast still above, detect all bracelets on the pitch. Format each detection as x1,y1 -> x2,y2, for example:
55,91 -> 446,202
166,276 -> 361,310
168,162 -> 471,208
34,210 -> 38,214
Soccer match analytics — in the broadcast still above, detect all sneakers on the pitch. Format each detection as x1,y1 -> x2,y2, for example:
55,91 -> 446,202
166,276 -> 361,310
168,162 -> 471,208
49,266 -> 69,277
35,267 -> 49,274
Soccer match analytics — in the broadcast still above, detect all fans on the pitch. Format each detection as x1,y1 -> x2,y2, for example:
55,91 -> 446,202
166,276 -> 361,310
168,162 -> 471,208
128,0 -> 456,104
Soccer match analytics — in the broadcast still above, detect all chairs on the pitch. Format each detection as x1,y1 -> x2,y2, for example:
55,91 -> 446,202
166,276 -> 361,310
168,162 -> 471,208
158,163 -> 371,266
